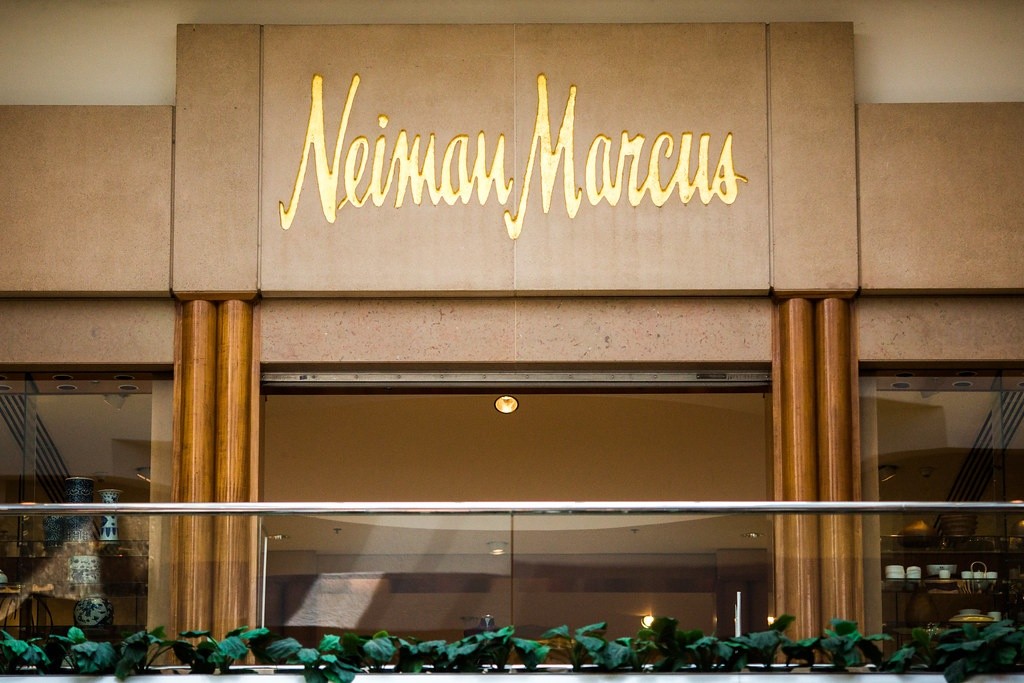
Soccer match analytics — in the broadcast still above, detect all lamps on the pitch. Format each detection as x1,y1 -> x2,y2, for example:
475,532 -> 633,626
104,394 -> 127,411
880,465 -> 896,481
136,468 -> 150,483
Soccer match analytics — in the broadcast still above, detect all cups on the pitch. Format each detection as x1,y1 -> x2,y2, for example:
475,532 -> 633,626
886,566 -> 921,578
940,570 -> 951,578
961,571 -> 998,578
988,611 -> 1002,622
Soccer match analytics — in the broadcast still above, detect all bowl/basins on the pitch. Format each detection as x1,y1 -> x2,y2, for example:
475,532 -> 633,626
926,565 -> 957,575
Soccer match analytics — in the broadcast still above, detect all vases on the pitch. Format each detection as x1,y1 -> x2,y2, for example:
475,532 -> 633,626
42,475 -> 124,630
